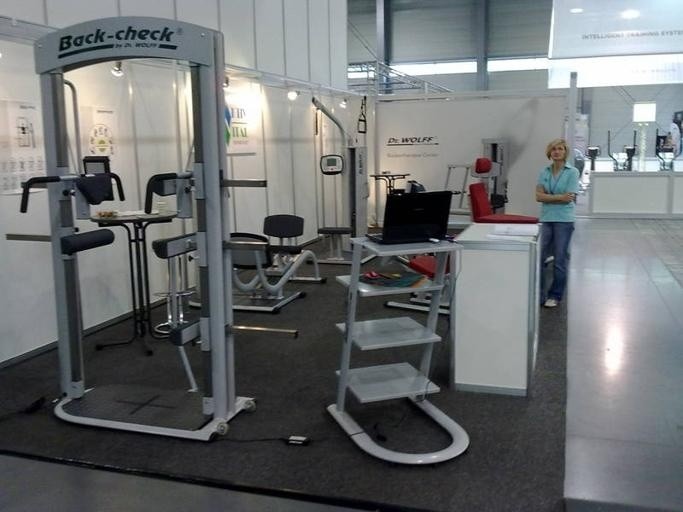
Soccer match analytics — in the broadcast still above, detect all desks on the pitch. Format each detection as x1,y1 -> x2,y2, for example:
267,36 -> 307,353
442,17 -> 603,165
588,171 -> 683,220
370,174 -> 408,194
91,210 -> 177,356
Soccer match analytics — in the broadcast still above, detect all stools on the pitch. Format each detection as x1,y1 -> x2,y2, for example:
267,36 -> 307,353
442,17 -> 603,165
318,227 -> 353,261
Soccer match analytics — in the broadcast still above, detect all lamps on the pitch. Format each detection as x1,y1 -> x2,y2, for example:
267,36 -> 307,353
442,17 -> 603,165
111,62 -> 123,76
223,77 -> 229,87
358,96 -> 366,132
289,91 -> 300,100
340,100 -> 347,108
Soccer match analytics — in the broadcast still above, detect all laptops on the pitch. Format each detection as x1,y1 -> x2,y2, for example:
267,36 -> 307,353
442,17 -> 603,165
365,191 -> 453,244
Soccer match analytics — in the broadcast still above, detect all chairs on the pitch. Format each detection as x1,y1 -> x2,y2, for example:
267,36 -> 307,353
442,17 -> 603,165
470,158 -> 538,223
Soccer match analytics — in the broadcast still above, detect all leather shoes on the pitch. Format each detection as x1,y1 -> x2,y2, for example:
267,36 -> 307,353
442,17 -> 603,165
545,297 -> 558,307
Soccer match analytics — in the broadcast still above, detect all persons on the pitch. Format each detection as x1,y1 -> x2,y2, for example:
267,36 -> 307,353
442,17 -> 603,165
534,138 -> 580,308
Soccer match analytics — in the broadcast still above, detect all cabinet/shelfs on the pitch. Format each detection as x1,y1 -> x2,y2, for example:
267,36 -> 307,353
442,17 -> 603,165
327,237 -> 471,466
